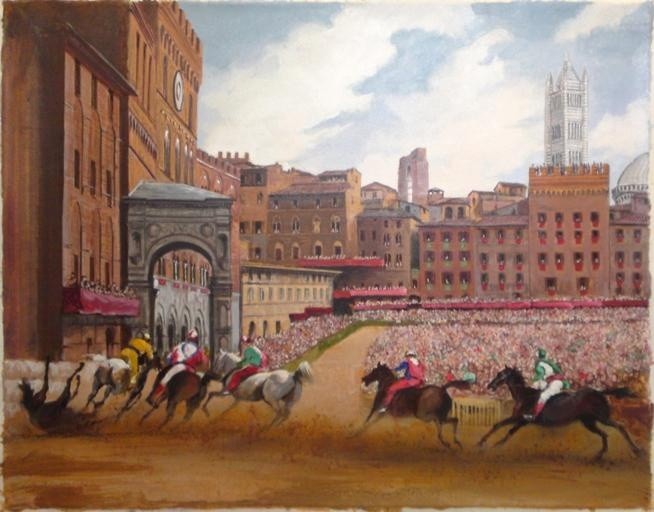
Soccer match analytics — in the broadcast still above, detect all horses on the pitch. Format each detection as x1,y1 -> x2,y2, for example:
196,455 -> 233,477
347,362 -> 471,453
16,348 -> 314,436
476,364 -> 640,462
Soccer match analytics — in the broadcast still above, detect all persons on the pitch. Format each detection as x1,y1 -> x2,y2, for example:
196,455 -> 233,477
149,328 -> 204,402
376,350 -> 426,415
222,333 -> 269,394
118,331 -> 155,389
522,347 -> 569,422
65,270 -> 136,299
242,252 -> 652,397
